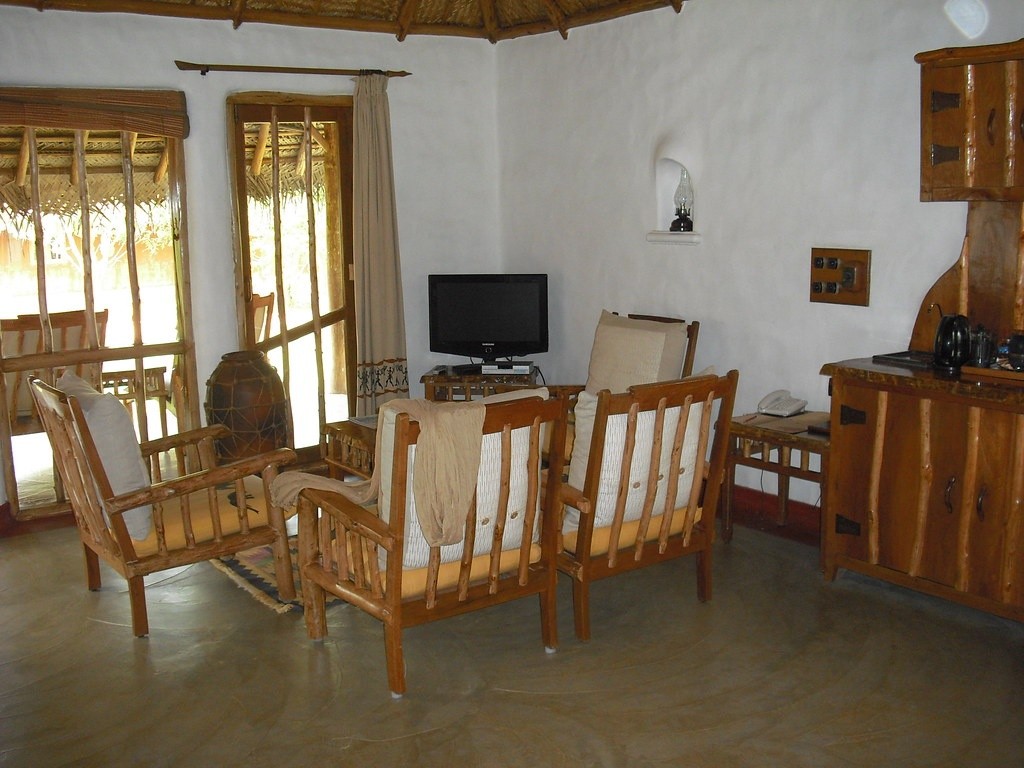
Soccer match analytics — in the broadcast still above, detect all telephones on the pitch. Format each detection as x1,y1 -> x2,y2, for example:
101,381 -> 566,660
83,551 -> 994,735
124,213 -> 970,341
757,389 -> 809,417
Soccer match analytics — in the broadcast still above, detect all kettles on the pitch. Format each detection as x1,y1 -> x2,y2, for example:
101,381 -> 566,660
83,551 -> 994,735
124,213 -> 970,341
933,314 -> 970,365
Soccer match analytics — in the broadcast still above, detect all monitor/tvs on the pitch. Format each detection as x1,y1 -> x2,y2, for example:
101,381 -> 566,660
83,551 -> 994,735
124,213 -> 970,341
428,273 -> 549,372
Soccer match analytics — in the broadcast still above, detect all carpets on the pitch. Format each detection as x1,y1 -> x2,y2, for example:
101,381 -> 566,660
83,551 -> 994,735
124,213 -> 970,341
208,534 -> 348,616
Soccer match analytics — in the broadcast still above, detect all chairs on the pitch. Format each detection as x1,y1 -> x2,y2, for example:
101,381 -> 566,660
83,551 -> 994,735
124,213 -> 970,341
296,395 -> 559,700
539,312 -> 700,467
254,291 -> 274,355
27,375 -> 298,637
540,369 -> 740,645
0,309 -> 109,504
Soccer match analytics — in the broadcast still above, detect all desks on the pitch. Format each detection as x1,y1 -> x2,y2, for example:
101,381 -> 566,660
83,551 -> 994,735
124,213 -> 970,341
711,410 -> 830,543
419,365 -> 539,401
320,413 -> 380,485
102,367 -> 168,444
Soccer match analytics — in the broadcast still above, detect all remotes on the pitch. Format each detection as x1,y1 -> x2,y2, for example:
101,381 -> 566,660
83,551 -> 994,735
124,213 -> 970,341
432,365 -> 447,374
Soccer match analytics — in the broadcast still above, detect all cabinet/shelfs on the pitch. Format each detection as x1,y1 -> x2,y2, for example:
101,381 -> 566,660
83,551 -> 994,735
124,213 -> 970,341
820,37 -> 1024,622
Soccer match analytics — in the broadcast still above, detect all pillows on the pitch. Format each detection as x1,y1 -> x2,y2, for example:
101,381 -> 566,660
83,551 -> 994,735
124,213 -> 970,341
378,386 -> 549,569
585,308 -> 688,397
560,366 -> 715,537
56,369 -> 152,541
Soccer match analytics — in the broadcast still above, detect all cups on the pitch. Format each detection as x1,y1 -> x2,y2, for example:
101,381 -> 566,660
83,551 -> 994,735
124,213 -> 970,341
969,324 -> 999,369
